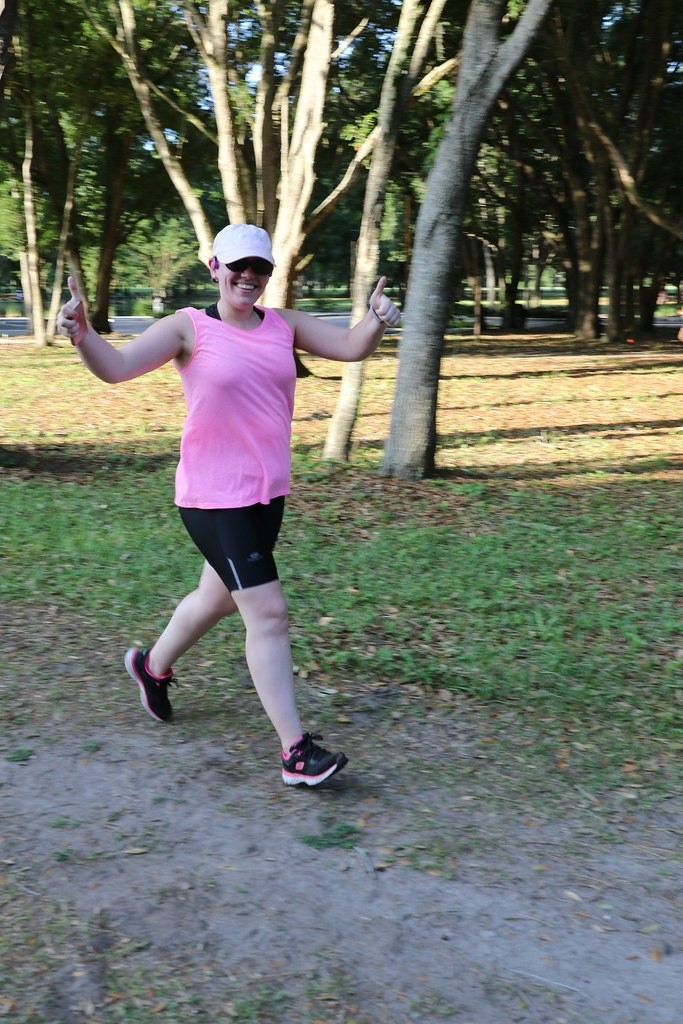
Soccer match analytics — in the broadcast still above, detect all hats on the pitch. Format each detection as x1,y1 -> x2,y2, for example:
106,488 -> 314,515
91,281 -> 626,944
212,224 -> 276,265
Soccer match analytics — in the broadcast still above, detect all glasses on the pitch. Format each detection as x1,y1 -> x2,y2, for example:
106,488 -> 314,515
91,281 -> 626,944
224,257 -> 273,276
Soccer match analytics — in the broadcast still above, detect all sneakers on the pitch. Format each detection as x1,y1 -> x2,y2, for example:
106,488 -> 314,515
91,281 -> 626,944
280,733 -> 345,787
124,646 -> 174,723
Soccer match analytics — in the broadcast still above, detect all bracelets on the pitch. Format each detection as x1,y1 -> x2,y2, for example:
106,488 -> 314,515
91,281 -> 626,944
368,305 -> 387,326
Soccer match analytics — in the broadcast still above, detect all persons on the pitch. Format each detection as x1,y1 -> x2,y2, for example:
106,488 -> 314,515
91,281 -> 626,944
49,224 -> 403,791
